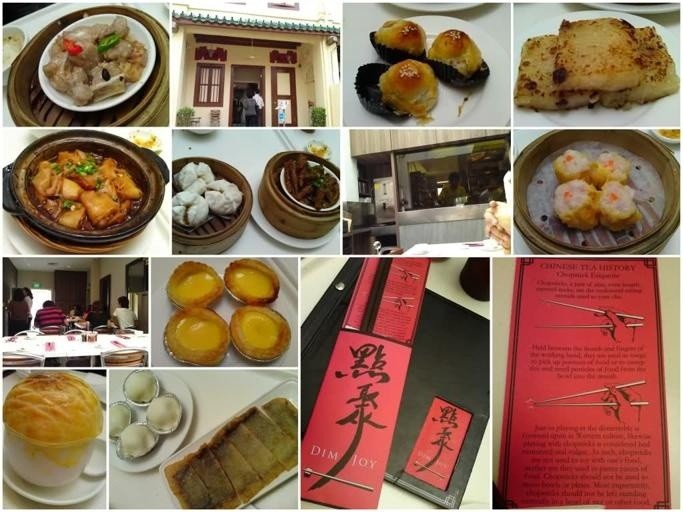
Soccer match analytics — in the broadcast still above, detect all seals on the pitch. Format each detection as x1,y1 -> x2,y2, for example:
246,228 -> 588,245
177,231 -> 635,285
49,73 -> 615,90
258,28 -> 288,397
172,161 -> 243,228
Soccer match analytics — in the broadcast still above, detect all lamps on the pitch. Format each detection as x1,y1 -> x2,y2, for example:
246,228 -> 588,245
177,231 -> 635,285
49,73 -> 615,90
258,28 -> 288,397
2,322 -> 147,367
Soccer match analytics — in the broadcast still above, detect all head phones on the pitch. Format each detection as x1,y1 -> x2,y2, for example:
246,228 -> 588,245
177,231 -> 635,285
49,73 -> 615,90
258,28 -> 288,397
359,258 -> 394,336
532,377 -> 649,407
535,298 -> 645,329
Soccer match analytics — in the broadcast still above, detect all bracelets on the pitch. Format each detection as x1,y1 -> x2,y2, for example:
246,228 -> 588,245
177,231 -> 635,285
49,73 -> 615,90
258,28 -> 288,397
4,129 -> 170,254
650,128 -> 680,145
2,27 -> 27,72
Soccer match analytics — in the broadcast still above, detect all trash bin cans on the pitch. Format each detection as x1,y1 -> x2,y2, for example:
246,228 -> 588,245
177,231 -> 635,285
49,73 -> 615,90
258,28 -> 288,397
436,171 -> 472,206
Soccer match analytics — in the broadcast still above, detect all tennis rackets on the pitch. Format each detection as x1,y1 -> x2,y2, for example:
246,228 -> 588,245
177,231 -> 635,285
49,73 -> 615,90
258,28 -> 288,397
163,259 -> 290,364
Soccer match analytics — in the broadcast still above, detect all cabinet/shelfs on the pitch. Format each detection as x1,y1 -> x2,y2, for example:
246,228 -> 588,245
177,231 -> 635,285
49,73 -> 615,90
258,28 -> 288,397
80,332 -> 98,343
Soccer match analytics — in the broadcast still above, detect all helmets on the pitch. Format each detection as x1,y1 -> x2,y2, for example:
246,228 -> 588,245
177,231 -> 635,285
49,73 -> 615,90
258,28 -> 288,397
581,4 -> 679,15
390,3 -> 484,13
108,369 -> 195,474
37,14 -> 158,113
514,11 -> 679,128
248,179 -> 337,251
2,370 -> 108,509
359,14 -> 512,127
158,377 -> 298,510
279,161 -> 340,211
162,257 -> 292,366
107,369 -> 184,461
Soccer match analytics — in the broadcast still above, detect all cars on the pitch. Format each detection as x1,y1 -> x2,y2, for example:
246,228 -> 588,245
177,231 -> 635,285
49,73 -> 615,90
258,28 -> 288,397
457,257 -> 489,302
43,340 -> 55,352
455,196 -> 464,206
482,239 -> 498,251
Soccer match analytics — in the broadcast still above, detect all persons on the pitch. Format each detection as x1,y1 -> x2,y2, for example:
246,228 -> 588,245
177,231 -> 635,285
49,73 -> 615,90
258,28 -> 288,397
251,88 -> 264,126
242,90 -> 259,126
483,199 -> 512,255
3,282 -> 140,337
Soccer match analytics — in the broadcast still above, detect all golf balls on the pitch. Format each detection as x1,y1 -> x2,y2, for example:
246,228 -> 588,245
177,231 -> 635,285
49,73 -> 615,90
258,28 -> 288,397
554,150 -> 642,232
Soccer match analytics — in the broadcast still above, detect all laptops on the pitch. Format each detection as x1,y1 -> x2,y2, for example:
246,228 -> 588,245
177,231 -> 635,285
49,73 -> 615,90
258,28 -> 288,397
378,58 -> 438,127
2,372 -> 103,445
428,29 -> 483,80
373,18 -> 426,56
